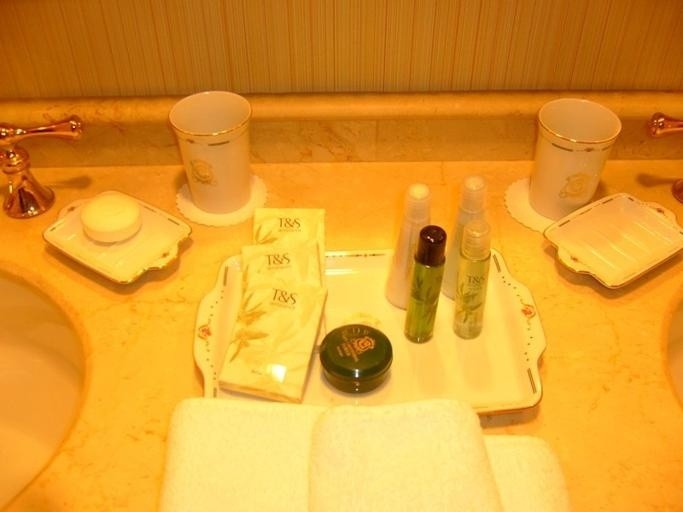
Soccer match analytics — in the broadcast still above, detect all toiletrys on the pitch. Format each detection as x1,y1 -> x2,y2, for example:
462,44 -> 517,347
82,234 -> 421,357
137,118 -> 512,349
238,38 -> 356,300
384,175 -> 493,345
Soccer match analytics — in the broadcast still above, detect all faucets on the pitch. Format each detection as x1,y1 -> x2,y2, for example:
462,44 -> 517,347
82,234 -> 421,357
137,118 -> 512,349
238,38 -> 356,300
648,112 -> 683,203
0,114 -> 84,220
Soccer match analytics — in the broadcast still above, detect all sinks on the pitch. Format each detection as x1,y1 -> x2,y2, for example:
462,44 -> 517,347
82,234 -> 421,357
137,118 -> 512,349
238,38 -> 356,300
0,257 -> 92,512
661,276 -> 683,409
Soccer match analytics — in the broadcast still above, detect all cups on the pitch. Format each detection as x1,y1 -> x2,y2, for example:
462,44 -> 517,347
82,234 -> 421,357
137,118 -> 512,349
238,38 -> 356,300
166,90 -> 252,214
529,97 -> 623,222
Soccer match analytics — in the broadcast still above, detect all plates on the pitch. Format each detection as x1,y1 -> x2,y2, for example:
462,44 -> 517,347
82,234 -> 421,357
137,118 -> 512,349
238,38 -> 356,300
192,247 -> 546,424
174,173 -> 269,229
543,190 -> 683,292
44,190 -> 191,288
504,176 -> 557,233
